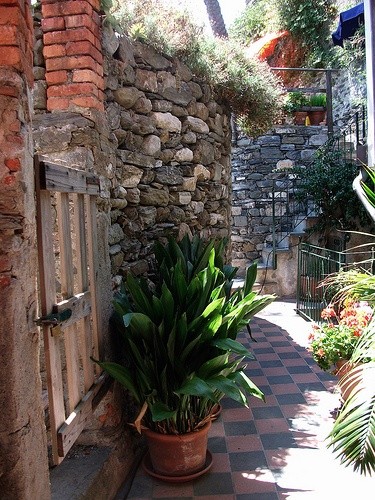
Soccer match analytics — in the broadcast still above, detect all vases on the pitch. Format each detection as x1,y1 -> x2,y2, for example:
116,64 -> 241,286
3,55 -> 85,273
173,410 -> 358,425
337,359 -> 363,402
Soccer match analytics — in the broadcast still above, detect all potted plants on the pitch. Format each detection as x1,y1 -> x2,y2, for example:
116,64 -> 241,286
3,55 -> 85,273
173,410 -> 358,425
286,93 -> 310,125
84,231 -> 276,481
307,92 -> 328,125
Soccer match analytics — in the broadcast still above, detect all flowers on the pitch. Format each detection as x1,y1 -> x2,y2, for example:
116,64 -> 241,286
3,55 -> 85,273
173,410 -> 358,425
308,300 -> 373,370
